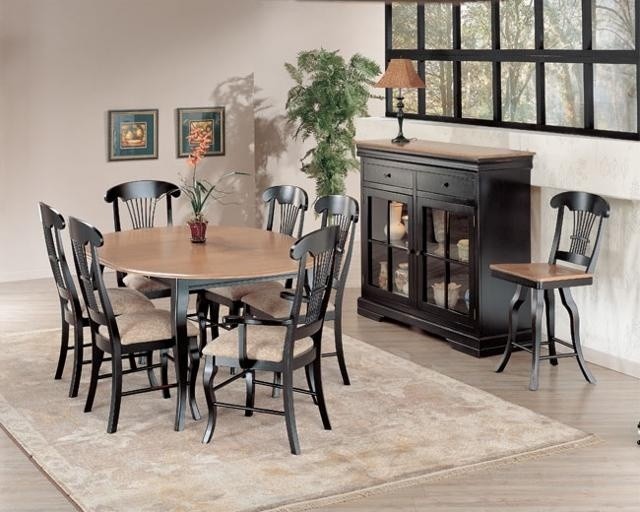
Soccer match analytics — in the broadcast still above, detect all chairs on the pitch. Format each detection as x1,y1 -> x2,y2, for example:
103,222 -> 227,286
195,184 -> 308,376
201,224 -> 341,456
67,214 -> 201,434
241,194 -> 359,398
40,201 -> 160,397
490,191 -> 611,392
104,179 -> 220,365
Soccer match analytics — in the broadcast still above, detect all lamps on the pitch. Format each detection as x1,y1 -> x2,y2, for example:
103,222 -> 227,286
374,59 -> 426,145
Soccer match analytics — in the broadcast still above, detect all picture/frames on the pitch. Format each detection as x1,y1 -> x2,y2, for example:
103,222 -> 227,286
108,108 -> 159,161
176,106 -> 225,158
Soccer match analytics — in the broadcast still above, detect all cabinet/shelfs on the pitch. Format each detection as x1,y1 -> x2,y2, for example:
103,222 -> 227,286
352,138 -> 534,359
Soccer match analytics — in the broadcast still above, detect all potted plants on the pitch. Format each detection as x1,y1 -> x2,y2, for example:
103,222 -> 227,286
287,42 -> 384,219
158,127 -> 253,243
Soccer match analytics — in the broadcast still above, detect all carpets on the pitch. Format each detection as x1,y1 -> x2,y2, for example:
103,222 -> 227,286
0,307 -> 599,512
